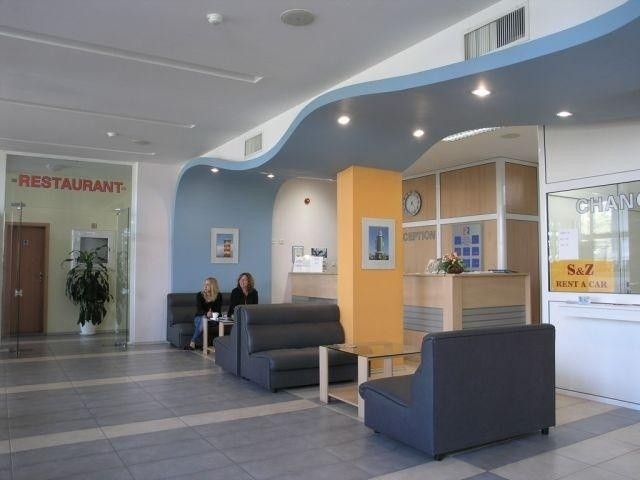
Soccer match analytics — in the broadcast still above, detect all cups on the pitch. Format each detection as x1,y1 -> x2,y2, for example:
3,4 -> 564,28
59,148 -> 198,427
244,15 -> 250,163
221,312 -> 228,318
212,313 -> 220,318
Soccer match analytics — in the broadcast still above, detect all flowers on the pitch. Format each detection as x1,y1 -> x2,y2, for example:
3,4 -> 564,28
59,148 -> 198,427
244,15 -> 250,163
435,252 -> 464,274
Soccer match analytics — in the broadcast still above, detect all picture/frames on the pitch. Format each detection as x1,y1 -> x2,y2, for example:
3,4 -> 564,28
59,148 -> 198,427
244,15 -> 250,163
362,218 -> 396,269
211,228 -> 240,264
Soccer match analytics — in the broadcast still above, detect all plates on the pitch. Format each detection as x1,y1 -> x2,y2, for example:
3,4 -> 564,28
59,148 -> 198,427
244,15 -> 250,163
210,318 -> 223,320
220,317 -> 230,319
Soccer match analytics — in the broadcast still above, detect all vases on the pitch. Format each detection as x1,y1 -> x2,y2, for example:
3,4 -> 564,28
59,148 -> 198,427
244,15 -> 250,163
448,266 -> 463,273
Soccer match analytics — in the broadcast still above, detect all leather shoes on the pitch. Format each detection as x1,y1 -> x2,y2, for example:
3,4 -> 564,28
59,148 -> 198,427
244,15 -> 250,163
188,341 -> 196,350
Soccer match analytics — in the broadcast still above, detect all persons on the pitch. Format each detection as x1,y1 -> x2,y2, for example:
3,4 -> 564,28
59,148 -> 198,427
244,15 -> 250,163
227,273 -> 259,316
183,277 -> 222,352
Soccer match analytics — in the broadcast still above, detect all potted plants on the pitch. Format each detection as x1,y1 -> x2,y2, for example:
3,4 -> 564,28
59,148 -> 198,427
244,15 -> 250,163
61,243 -> 114,335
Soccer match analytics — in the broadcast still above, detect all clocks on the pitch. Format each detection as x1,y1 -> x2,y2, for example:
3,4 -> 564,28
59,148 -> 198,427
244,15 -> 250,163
403,191 -> 422,217
